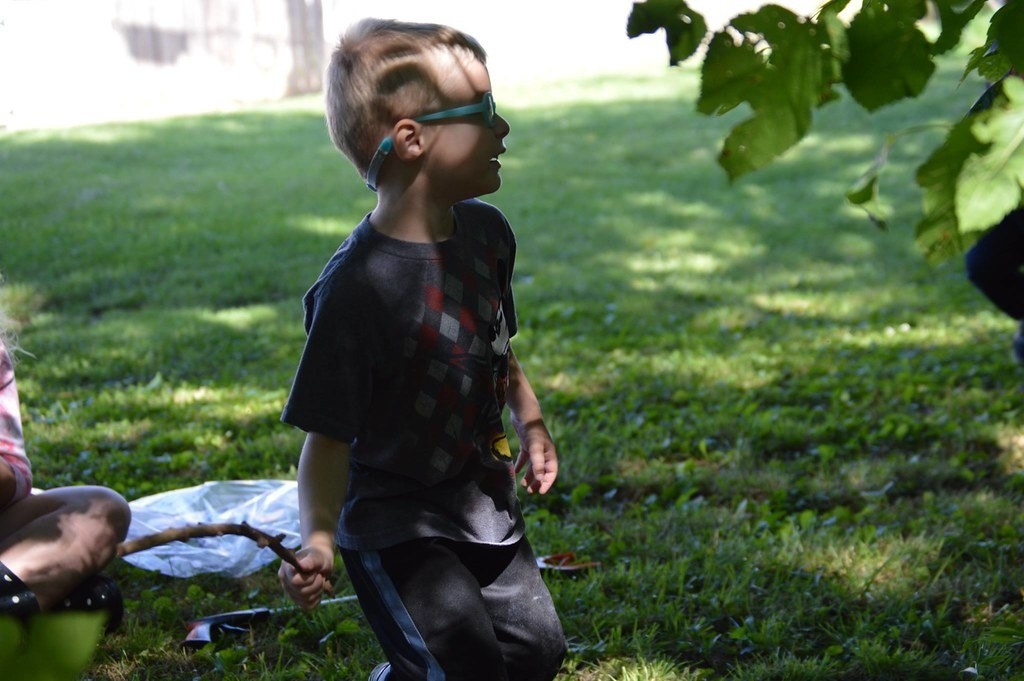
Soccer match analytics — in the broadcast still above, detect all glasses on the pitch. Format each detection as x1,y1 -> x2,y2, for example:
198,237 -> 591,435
366,90 -> 497,192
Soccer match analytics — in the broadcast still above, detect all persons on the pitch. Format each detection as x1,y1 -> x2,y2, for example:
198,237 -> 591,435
276,15 -> 569,681
0,321 -> 132,617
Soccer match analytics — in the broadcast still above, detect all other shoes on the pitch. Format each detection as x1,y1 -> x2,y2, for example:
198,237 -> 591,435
0,561 -> 40,617
55,575 -> 122,635
